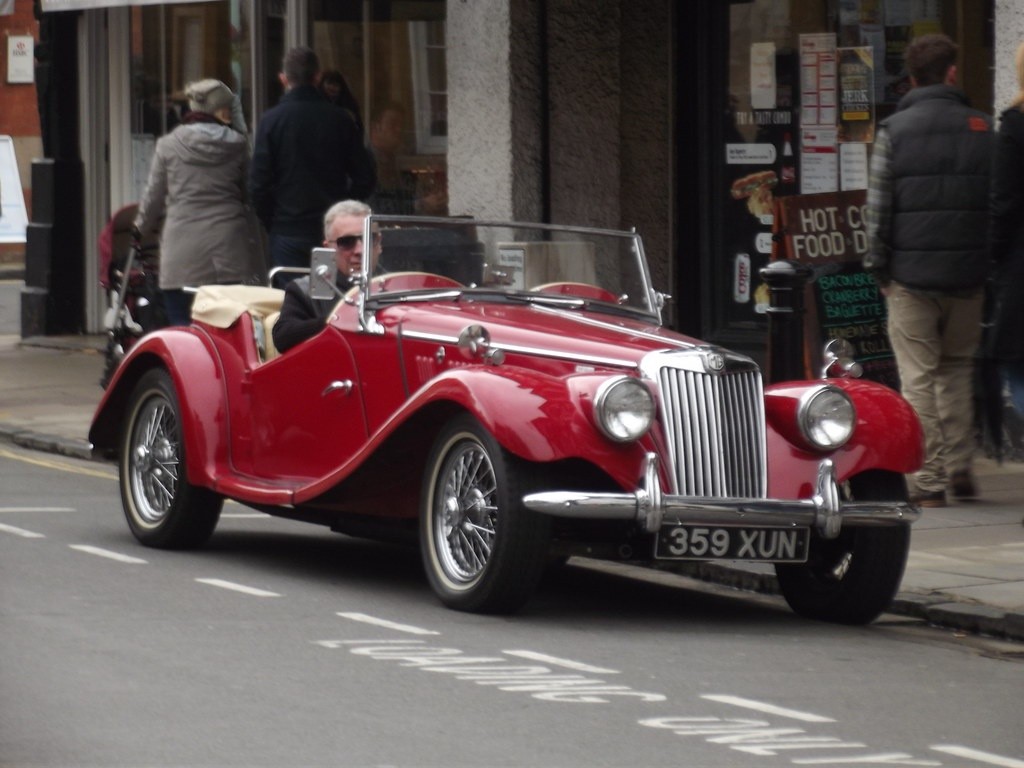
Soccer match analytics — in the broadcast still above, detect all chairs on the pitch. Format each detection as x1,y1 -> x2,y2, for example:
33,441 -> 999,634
264,312 -> 282,362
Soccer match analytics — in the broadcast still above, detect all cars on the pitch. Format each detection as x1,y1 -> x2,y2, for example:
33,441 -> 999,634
88,210 -> 929,627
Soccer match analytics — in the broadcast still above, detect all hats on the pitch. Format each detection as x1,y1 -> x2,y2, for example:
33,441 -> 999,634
182,79 -> 237,115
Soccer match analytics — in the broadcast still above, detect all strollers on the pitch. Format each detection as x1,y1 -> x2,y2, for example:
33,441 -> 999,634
88,201 -> 195,393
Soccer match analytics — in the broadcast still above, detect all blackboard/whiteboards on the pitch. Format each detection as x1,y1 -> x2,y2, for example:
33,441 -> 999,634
802,261 -> 911,503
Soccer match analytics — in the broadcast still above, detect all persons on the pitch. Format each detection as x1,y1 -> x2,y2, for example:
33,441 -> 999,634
866,34 -> 997,507
130,78 -> 269,292
997,46 -> 1024,442
272,200 -> 383,354
251,47 -> 378,288
320,70 -> 363,141
366,101 -> 405,211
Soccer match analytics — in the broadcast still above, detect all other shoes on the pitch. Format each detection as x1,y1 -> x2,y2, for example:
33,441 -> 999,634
949,469 -> 980,496
907,479 -> 947,509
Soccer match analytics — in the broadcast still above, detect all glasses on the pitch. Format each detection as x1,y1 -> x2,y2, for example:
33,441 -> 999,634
326,232 -> 382,249
